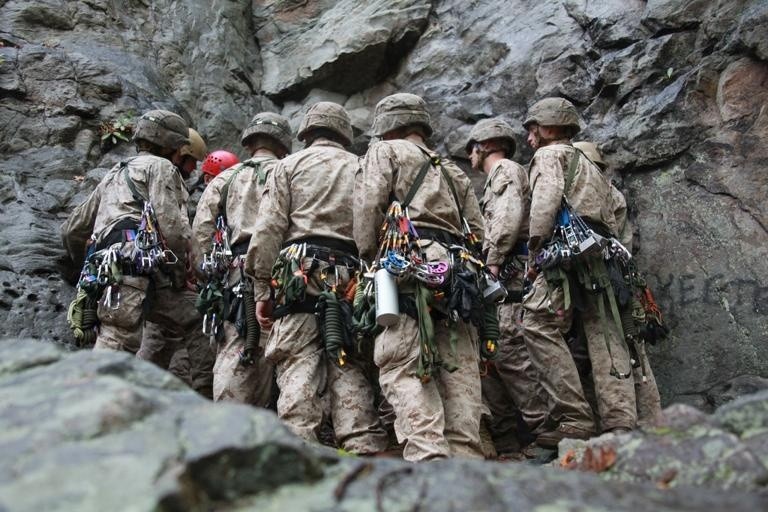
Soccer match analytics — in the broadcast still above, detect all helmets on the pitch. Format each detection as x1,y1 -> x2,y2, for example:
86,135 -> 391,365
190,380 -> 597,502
242,112 -> 292,153
297,101 -> 354,146
465,119 -> 517,159
133,110 -> 239,177
523,98 -> 580,138
364,93 -> 433,140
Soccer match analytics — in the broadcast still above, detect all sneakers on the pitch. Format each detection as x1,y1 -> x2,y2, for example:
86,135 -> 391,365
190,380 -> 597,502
520,422 -> 590,459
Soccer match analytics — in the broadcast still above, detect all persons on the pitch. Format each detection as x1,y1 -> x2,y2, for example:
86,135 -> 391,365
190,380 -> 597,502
59,93 -> 662,464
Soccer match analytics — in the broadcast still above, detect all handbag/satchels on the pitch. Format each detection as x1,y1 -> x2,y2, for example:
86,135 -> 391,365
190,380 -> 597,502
97,275 -> 150,332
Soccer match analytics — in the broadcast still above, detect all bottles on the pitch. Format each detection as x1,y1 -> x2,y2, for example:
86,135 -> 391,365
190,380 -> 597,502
373,268 -> 401,329
